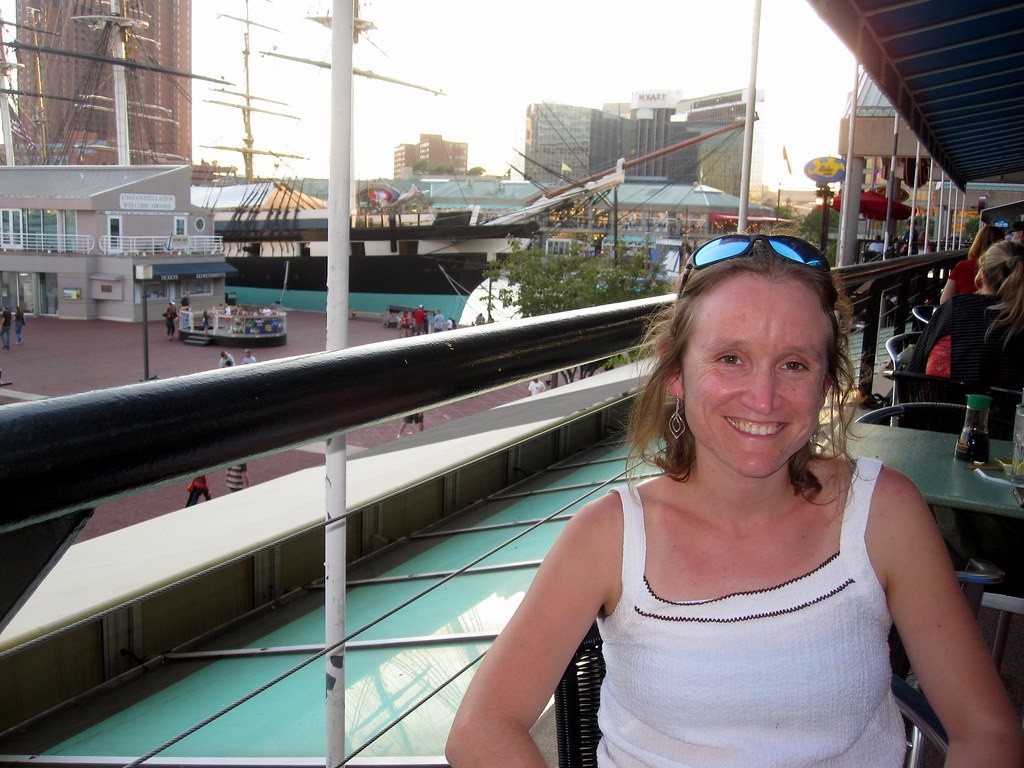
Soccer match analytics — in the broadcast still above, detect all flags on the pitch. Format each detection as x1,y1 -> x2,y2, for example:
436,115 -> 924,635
782,145 -> 792,175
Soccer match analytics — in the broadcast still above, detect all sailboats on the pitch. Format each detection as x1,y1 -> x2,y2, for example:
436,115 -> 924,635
0,0 -> 760,295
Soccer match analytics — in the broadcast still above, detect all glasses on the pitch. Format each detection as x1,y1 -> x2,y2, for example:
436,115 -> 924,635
677,236 -> 829,302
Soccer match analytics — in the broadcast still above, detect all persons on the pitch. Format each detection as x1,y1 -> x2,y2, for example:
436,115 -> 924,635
0,305 -> 12,349
162,292 -> 272,341
218,351 -> 235,368
185,473 -> 211,508
226,462 -> 249,493
222,361 -> 231,368
401,304 -> 456,338
867,220 -> 1024,442
527,378 -> 547,397
12,305 -> 26,344
445,226 -> 1024,768
241,349 -> 256,365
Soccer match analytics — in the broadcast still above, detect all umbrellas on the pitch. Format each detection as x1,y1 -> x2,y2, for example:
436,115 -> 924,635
832,190 -> 917,239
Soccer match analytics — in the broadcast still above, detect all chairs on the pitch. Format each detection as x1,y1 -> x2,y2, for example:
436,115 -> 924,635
550,306 -> 1024,768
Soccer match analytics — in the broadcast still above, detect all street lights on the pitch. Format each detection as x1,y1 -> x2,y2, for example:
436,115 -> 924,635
135,264 -> 153,379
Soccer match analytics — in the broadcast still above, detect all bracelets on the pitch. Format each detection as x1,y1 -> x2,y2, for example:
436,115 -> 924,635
396,412 -> 424,440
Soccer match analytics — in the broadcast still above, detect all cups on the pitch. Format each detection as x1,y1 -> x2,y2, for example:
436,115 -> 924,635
1013,404 -> 1024,480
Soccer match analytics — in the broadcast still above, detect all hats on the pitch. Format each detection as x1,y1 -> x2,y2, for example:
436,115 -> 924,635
1005,223 -> 1024,235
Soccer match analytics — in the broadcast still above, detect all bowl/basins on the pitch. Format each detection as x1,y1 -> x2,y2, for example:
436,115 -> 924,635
996,457 -> 1012,475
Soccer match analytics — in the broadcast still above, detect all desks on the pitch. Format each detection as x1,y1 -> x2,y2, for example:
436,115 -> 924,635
818,420 -> 1024,560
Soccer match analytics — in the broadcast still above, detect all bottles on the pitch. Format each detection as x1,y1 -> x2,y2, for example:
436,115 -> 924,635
954,394 -> 992,463
